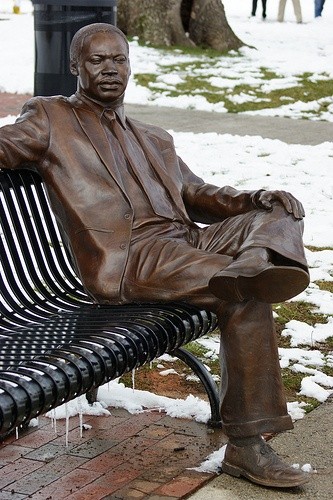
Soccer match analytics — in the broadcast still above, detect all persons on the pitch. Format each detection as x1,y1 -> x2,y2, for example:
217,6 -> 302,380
276,0 -> 304,24
312,0 -> 326,22
1,23 -> 312,489
247,0 -> 270,22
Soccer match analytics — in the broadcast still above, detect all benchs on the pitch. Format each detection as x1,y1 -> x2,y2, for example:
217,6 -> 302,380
1,166 -> 224,438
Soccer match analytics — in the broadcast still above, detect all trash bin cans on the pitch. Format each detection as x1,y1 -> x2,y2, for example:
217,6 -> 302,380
33,0 -> 121,95
